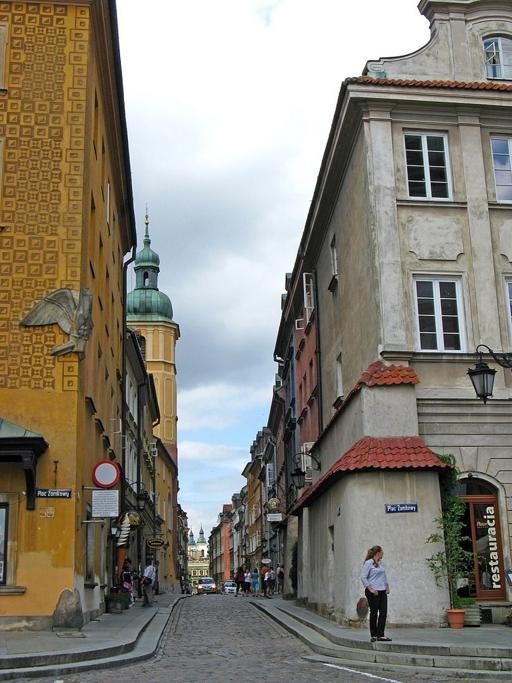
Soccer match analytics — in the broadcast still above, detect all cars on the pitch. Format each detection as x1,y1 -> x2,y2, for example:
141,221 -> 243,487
223,579 -> 238,595
196,576 -> 217,593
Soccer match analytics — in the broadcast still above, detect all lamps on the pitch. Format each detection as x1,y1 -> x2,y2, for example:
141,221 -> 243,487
121,481 -> 148,511
290,452 -> 321,489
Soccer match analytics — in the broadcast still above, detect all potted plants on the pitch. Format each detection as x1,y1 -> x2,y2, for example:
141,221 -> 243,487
461,597 -> 483,626
108,592 -> 130,614
424,452 -> 472,630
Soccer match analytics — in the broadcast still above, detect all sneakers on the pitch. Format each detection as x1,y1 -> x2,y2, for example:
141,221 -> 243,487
129,599 -> 158,608
370,637 -> 393,643
234,591 -> 281,598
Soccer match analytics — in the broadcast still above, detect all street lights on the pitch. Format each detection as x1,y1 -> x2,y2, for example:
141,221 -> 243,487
110,481 -> 147,591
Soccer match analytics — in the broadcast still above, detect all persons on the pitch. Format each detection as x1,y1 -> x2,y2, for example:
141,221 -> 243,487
360,546 -> 392,642
140,559 -> 156,607
120,565 -> 135,607
234,563 -> 284,599
